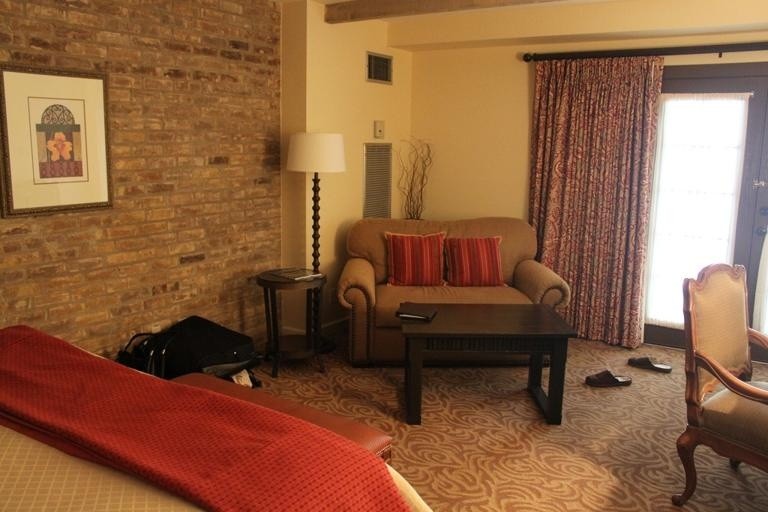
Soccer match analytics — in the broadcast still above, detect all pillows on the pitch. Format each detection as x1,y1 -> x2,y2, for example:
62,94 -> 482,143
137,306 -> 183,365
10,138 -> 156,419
445,236 -> 507,288
384,232 -> 449,287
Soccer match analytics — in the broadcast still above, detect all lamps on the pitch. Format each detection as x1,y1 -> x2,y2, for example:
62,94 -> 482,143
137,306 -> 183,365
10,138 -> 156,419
286,133 -> 344,352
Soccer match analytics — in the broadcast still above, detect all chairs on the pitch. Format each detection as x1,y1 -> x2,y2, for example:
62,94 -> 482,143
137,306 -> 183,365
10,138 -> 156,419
672,263 -> 768,507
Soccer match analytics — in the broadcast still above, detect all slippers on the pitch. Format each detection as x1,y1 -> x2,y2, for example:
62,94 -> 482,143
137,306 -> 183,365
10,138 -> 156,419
627,354 -> 672,373
586,369 -> 633,387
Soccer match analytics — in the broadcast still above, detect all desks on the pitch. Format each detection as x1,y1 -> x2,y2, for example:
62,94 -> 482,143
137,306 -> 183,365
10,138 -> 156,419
401,304 -> 577,425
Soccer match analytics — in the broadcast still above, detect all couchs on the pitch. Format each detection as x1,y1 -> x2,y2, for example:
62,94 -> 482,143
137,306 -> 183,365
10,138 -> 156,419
337,218 -> 572,367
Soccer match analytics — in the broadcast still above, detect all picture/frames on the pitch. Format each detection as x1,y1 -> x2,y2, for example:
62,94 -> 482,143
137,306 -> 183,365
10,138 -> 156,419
0,65 -> 113,218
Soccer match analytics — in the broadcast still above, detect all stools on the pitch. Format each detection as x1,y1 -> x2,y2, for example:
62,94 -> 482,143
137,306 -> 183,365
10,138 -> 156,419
170,374 -> 394,461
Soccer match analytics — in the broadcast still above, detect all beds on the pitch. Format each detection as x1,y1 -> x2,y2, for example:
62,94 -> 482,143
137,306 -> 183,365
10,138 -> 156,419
0,325 -> 436,511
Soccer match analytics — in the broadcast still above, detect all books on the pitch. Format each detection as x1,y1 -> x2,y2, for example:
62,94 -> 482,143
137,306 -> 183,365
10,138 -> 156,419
394,301 -> 439,323
268,267 -> 322,282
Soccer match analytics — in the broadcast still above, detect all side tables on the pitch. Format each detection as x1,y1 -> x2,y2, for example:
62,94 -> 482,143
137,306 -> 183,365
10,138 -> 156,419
257,268 -> 327,377
